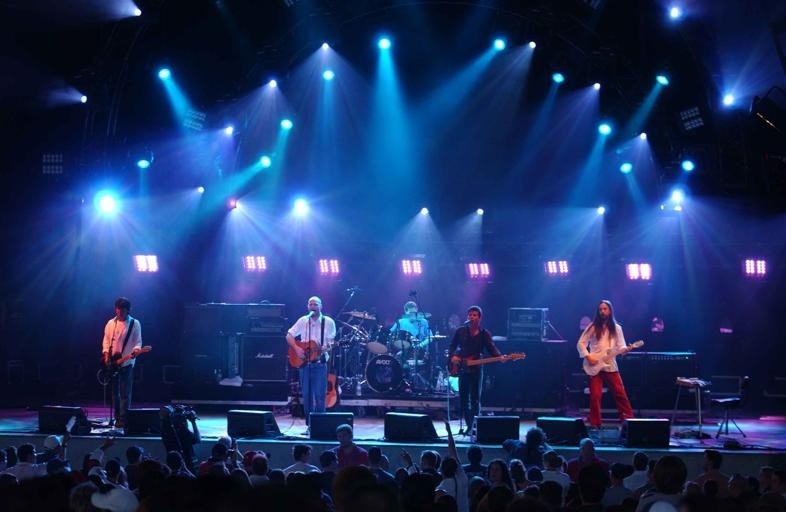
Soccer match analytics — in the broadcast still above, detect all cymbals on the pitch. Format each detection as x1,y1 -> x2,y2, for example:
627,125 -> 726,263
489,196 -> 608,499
425,335 -> 445,338
346,311 -> 375,319
400,313 -> 431,318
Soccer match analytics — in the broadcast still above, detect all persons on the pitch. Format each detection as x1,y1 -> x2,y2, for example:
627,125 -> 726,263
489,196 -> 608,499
1,424 -> 786,511
649,317 -> 665,335
159,405 -> 200,476
388,302 -> 434,359
448,305 -> 507,435
286,296 -> 336,436
102,297 -> 142,428
576,300 -> 634,431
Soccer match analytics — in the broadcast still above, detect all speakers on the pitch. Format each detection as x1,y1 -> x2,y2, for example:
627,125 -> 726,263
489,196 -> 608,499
124,407 -> 162,437
536,416 -> 589,447
239,333 -> 288,383
383,412 -> 438,442
620,418 -> 670,447
308,412 -> 353,441
39,406 -> 87,434
470,416 -> 520,444
228,409 -> 283,439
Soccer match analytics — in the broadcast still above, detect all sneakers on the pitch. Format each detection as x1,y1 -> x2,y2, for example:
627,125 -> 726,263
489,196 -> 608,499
300,427 -> 308,435
115,418 -> 123,428
464,428 -> 471,436
589,424 -> 597,431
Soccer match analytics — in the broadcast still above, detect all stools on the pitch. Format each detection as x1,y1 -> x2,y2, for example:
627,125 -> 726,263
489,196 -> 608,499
669,380 -> 712,438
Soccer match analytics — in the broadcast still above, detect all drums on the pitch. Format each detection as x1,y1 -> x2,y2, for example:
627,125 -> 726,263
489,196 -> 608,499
366,324 -> 391,353
364,354 -> 402,394
393,330 -> 412,349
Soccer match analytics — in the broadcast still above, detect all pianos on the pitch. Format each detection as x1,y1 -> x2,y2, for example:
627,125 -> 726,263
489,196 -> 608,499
620,351 -> 696,360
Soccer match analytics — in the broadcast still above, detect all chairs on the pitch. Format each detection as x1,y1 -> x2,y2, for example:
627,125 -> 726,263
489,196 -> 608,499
711,376 -> 749,438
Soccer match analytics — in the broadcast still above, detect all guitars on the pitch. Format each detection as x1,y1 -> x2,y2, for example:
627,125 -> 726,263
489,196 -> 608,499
583,341 -> 644,376
324,347 -> 339,409
97,347 -> 152,385
289,340 -> 330,368
449,353 -> 526,376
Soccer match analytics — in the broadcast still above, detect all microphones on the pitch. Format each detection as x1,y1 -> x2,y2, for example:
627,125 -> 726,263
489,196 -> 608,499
306,310 -> 316,319
114,315 -> 121,323
409,290 -> 417,296
347,288 -> 364,294
464,320 -> 474,326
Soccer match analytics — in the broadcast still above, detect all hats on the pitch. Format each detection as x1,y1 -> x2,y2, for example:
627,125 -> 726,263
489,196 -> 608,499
198,462 -> 207,475
125,446 -> 144,464
242,452 -> 256,467
43,435 -> 64,450
47,458 -> 68,472
